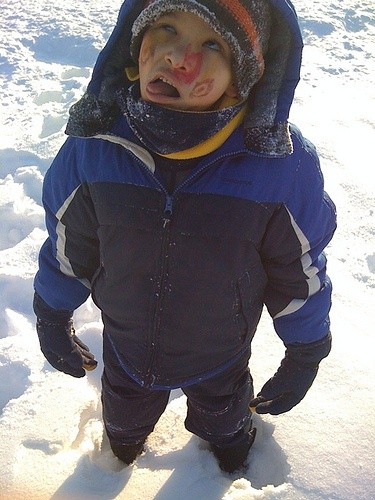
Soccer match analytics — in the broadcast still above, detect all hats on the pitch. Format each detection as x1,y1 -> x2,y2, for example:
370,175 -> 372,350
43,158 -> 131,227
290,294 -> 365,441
129,0 -> 274,101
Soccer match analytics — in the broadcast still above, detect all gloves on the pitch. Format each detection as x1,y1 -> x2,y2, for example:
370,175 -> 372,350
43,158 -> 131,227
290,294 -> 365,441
233,331 -> 333,415
33,292 -> 98,378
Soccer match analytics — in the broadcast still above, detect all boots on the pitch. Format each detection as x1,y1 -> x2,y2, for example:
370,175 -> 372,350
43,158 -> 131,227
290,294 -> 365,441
102,424 -> 146,464
209,423 -> 257,473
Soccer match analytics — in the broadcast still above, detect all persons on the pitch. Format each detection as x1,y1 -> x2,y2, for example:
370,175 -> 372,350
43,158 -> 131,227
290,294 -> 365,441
33,0 -> 339,472
